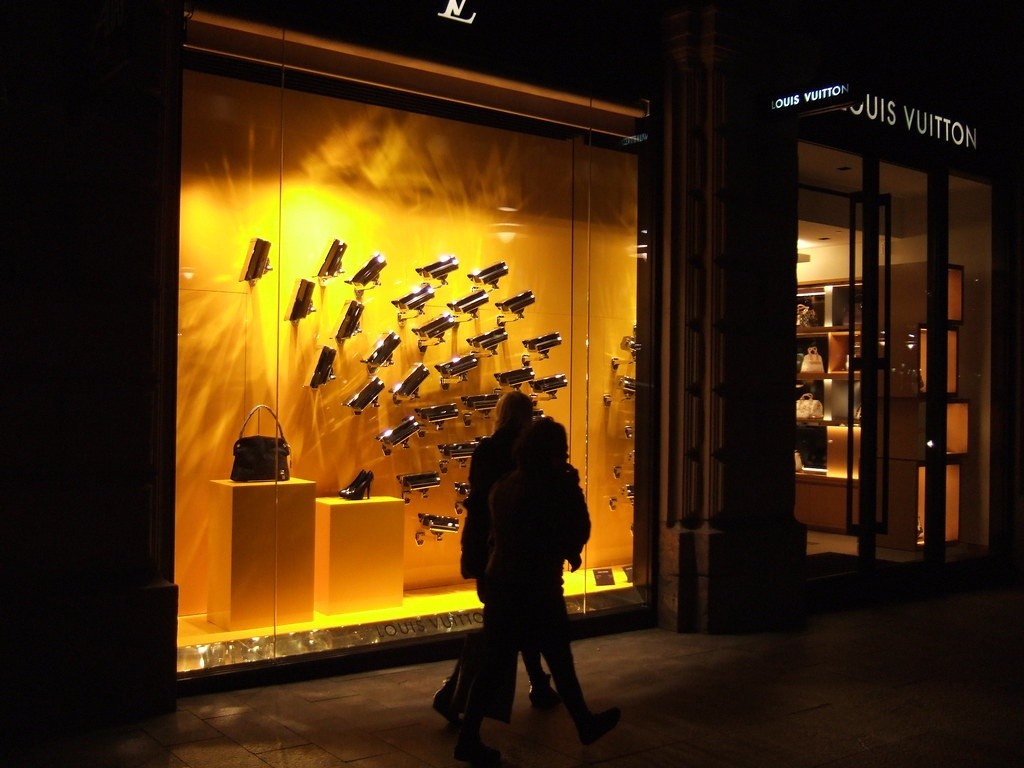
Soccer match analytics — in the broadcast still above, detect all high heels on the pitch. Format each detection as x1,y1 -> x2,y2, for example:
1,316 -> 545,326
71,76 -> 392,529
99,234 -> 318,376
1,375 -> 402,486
339,469 -> 374,500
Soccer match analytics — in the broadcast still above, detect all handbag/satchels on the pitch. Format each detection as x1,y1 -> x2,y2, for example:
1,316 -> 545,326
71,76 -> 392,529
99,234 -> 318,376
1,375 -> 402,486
797,300 -> 819,327
841,294 -> 862,326
845,342 -> 863,371
231,405 -> 290,481
796,393 -> 824,420
800,347 -> 824,373
794,440 -> 810,471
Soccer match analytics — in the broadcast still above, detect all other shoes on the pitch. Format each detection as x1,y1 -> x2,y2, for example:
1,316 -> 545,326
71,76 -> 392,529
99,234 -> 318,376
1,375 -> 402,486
454,741 -> 501,764
576,707 -> 621,745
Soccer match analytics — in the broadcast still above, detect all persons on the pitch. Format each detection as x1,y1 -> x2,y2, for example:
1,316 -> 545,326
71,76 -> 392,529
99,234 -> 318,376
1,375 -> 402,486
453,417 -> 622,768
431,392 -> 562,722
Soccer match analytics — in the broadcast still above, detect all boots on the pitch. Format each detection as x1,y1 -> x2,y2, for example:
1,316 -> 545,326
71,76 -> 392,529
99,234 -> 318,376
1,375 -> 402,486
528,674 -> 563,707
433,680 -> 460,724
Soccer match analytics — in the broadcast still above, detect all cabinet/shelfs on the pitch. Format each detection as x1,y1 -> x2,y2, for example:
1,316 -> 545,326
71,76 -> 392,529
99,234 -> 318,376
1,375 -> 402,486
877,327 -> 960,398
795,276 -> 864,534
875,460 -> 962,549
878,398 -> 972,460
878,261 -> 965,326
